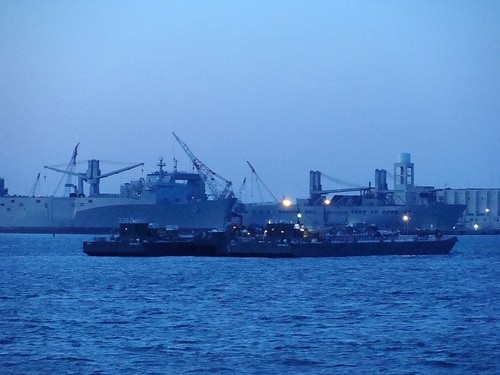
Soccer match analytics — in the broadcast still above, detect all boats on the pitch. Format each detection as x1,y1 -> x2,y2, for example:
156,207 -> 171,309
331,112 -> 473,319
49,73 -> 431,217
82,217 -> 458,257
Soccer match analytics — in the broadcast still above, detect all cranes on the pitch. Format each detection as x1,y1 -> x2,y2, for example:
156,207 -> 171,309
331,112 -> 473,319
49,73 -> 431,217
235,177 -> 248,204
27,172 -> 41,198
171,132 -> 232,200
50,141 -> 81,198
43,158 -> 144,199
247,161 -> 278,204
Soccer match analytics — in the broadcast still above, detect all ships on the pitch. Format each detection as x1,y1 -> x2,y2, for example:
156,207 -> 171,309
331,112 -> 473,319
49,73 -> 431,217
230,194 -> 468,235
0,131 -> 240,235
